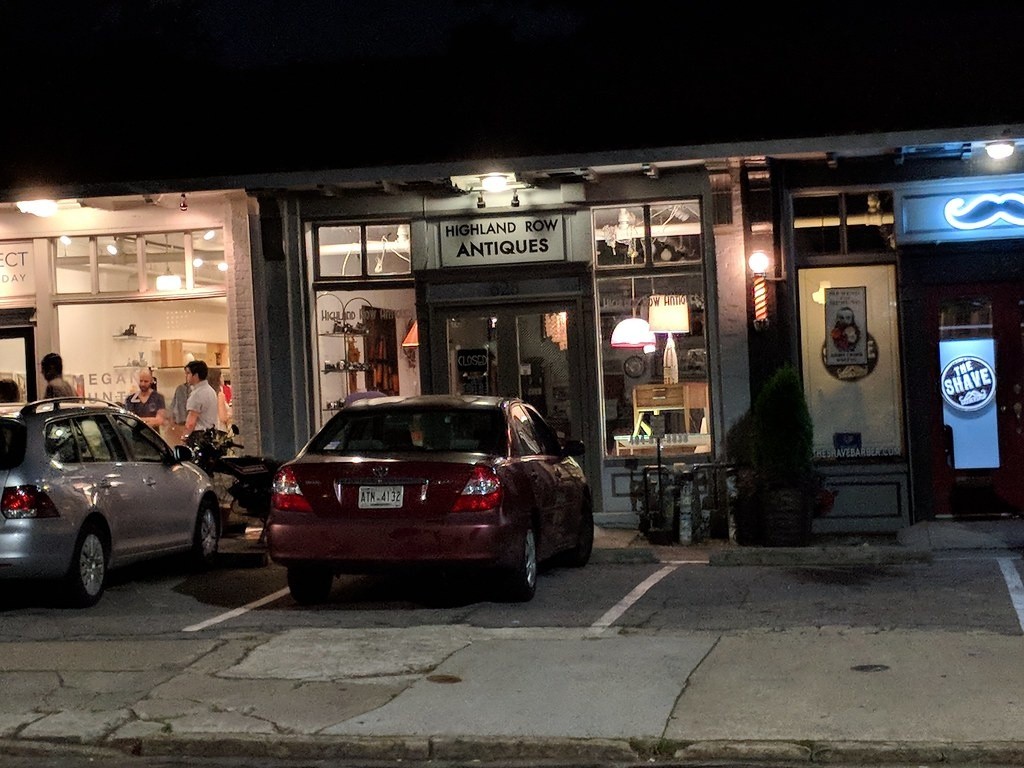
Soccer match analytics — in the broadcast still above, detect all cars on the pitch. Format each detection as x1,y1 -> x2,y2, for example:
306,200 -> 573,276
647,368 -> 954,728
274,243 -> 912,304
265,395 -> 594,601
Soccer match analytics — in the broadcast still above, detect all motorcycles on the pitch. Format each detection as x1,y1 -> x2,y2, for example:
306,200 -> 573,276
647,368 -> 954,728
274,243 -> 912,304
186,425 -> 284,545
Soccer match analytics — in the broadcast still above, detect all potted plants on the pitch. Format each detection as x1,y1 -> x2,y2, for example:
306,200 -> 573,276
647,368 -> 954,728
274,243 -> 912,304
181,424 -> 242,537
721,362 -> 814,546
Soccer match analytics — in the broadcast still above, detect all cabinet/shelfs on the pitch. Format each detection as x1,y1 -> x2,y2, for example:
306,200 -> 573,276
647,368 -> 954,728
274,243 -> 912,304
632,382 -> 710,434
318,332 -> 368,411
113,335 -> 156,372
158,339 -> 229,369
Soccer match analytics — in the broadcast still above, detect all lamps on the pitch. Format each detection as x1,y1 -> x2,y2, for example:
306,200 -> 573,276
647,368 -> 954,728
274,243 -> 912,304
476,190 -> 486,209
510,188 -> 520,207
402,319 -> 418,347
106,237 -> 120,254
156,234 -> 181,295
610,279 -> 656,348
748,250 -> 769,333
647,293 -> 690,385
179,191 -> 187,211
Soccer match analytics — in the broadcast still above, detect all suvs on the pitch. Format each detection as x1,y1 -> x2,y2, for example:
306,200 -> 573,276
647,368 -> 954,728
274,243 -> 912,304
1,398 -> 222,610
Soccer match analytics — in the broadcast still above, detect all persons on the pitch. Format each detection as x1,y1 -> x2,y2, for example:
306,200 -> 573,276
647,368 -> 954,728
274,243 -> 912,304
41,353 -> 74,399
207,368 -> 232,440
123,368 -> 166,435
179,361 -> 218,451
0,379 -> 20,403
